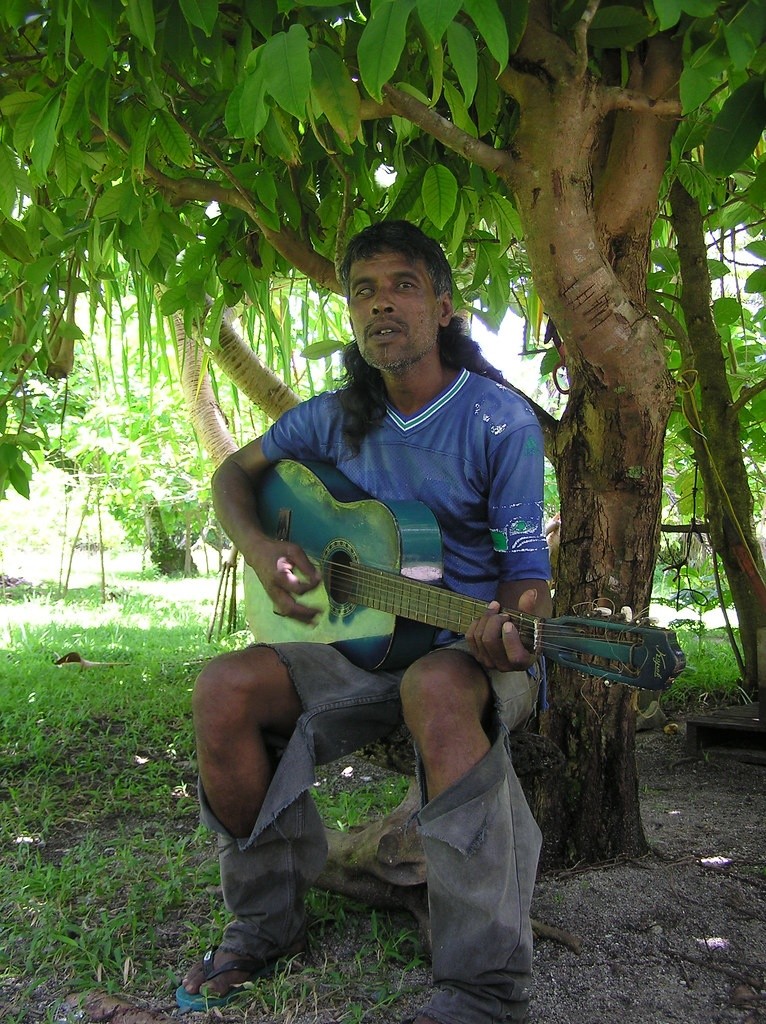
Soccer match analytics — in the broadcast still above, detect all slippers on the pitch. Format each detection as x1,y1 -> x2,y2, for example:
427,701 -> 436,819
176,944 -> 306,1008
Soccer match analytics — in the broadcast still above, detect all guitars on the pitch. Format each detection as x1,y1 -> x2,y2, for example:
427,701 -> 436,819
239,456 -> 688,692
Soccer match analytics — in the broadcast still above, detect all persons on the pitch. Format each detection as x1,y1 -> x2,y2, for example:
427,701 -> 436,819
174,224 -> 557,1024
